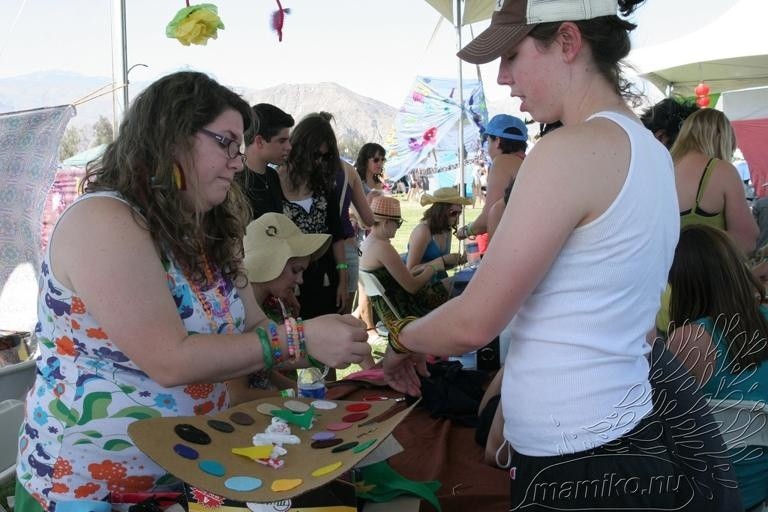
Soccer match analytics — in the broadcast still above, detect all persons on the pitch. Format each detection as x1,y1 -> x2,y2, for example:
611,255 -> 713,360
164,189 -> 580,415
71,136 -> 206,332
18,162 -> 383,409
14,1 -> 768,512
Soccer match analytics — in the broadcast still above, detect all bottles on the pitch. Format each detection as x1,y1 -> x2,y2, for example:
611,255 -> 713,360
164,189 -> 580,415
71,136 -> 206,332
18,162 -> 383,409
463,234 -> 481,269
296,367 -> 327,400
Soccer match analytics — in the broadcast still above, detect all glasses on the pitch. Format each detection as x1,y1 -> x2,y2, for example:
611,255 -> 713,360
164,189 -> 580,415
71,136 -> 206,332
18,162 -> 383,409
195,126 -> 247,165
367,157 -> 387,163
392,220 -> 404,227
449,209 -> 462,217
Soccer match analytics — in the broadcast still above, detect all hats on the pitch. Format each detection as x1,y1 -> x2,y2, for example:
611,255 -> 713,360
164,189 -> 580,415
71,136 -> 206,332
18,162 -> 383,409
370,196 -> 407,221
485,114 -> 528,142
420,188 -> 472,206
456,0 -> 618,63
243,212 -> 332,283
